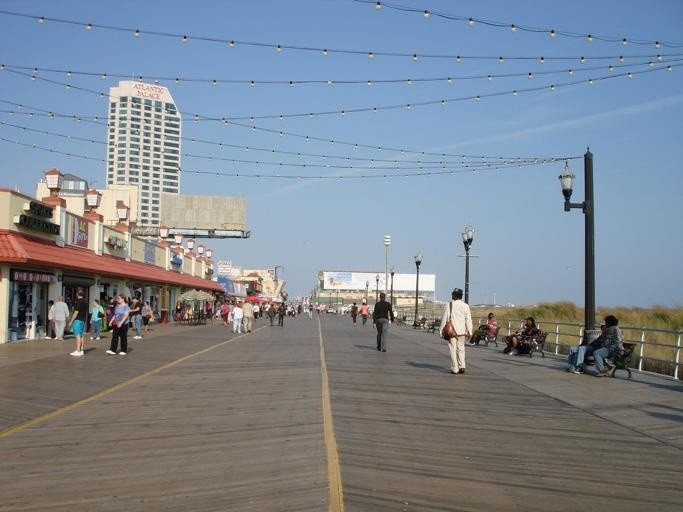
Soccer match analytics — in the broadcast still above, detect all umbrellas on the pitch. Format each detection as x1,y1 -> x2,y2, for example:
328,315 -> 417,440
176,289 -> 207,310
197,290 -> 216,311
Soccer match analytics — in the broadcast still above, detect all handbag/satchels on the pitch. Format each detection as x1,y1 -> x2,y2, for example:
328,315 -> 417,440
442,322 -> 457,340
98,312 -> 105,318
146,315 -> 152,320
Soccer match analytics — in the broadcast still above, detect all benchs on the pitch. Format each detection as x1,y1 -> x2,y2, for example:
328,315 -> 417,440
412,316 -> 427,330
501,331 -> 549,360
575,341 -> 638,381
397,315 -> 407,326
425,318 -> 441,334
473,325 -> 501,348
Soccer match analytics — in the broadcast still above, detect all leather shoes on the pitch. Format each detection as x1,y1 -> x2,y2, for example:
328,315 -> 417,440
459,368 -> 465,373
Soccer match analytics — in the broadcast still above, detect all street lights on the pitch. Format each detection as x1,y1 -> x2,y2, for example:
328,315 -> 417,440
383,235 -> 391,301
461,224 -> 475,305
376,274 -> 380,302
413,251 -> 423,326
557,145 -> 596,346
389,266 -> 395,310
336,288 -> 339,305
365,280 -> 369,306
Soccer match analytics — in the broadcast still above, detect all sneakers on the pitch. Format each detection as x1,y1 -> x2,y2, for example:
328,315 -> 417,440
45,335 -> 143,357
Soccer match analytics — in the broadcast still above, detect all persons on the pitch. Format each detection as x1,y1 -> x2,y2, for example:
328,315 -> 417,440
44,296 -> 153,341
350,302 -> 357,324
174,300 -> 324,327
567,320 -> 608,375
242,301 -> 253,333
67,287 -> 86,357
592,315 -> 623,376
359,303 -> 368,324
105,294 -> 130,356
504,317 -> 537,358
437,287 -> 473,374
465,313 -> 496,346
372,293 -> 393,353
230,303 -> 243,333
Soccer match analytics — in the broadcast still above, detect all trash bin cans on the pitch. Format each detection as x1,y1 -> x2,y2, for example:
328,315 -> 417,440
161,311 -> 168,323
585,330 -> 602,361
